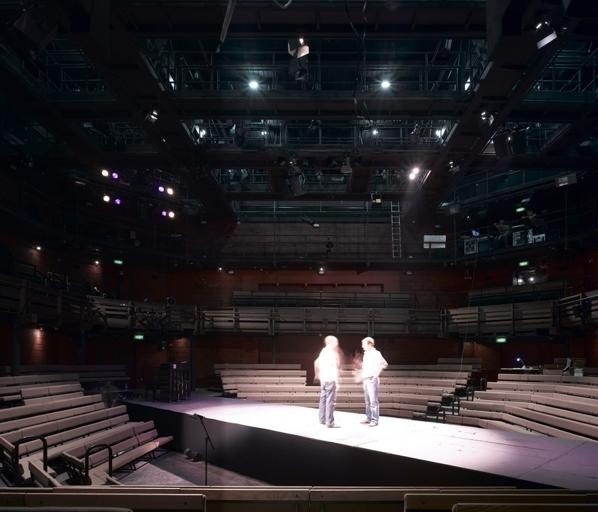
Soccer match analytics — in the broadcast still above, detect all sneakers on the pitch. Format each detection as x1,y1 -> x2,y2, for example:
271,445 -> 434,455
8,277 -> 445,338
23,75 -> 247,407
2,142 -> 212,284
332,423 -> 340,427
361,418 -> 369,423
368,421 -> 376,426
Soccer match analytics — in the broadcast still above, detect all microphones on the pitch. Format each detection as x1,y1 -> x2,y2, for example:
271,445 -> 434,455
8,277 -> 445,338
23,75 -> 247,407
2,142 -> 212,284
194,413 -> 203,419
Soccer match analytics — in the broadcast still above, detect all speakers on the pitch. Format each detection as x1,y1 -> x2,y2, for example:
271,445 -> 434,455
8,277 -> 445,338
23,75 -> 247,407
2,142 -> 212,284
159,362 -> 189,401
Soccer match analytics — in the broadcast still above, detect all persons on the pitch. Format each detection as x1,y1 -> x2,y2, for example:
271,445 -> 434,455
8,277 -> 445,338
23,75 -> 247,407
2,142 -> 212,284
360,336 -> 388,427
314,335 -> 342,428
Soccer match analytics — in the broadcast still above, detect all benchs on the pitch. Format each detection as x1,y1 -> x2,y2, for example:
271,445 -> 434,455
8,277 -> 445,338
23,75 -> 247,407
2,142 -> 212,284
3,279 -> 596,338
2,486 -> 596,510
2,363 -> 175,485
214,355 -> 596,440
